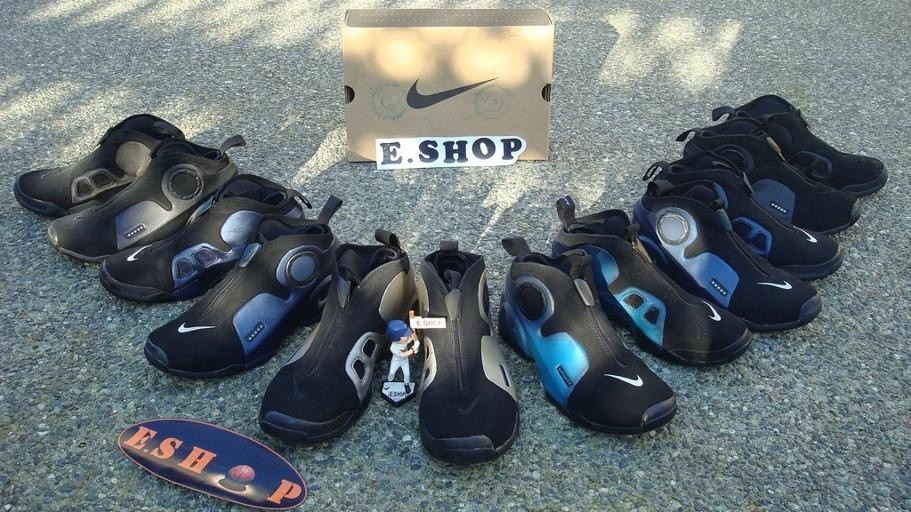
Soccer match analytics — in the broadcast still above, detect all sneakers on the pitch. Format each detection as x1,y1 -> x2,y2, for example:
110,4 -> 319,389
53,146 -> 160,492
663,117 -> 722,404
418,239 -> 520,463
675,117 -> 862,235
712,95 -> 887,198
552,195 -> 752,367
640,151 -> 843,281
499,237 -> 677,434
258,228 -> 419,443
632,179 -> 822,333
13,113 -> 185,217
144,195 -> 343,378
98,173 -> 312,303
47,134 -> 247,262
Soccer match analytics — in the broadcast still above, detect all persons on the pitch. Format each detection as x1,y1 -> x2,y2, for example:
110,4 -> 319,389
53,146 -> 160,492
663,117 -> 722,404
379,309 -> 446,407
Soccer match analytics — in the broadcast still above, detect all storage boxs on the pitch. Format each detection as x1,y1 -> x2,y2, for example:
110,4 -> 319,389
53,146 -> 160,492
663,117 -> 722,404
338,5 -> 561,172
